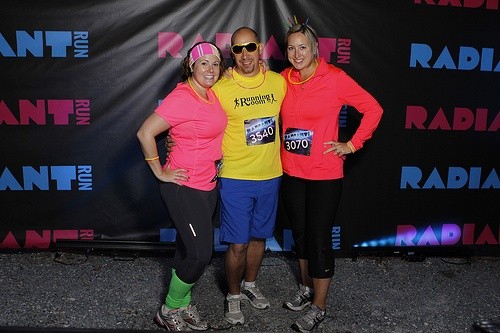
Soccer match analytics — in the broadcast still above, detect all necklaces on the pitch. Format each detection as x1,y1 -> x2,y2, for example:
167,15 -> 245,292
186,77 -> 215,104
232,64 -> 266,90
287,59 -> 319,85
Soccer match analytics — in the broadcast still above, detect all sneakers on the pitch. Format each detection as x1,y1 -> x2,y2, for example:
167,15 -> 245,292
285,283 -> 314,310
179,302 -> 210,330
224,292 -> 244,325
155,304 -> 192,333
291,305 -> 326,333
241,280 -> 270,310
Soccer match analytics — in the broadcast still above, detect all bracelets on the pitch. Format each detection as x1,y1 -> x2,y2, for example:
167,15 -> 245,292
144,156 -> 160,161
346,141 -> 356,153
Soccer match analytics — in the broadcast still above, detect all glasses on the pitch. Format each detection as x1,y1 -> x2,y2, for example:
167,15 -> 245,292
231,42 -> 260,55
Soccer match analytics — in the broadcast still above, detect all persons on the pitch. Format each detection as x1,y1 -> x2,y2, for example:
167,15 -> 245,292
164,26 -> 286,327
135,42 -> 266,333
219,22 -> 383,333
